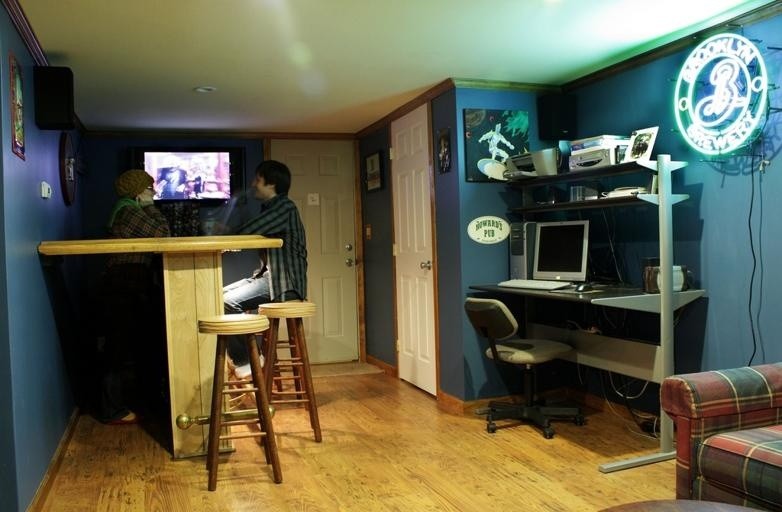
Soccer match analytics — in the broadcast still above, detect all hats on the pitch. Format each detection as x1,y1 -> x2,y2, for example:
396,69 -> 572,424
113,170 -> 154,200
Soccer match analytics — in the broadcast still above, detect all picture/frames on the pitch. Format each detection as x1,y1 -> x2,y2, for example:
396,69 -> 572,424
363,151 -> 385,194
437,127 -> 451,175
8,48 -> 26,163
59,131 -> 78,207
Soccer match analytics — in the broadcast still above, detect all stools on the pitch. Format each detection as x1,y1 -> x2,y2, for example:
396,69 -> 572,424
198,314 -> 282,491
258,302 -> 321,447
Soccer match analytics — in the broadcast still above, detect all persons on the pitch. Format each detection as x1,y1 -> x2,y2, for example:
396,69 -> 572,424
97,169 -> 172,425
146,150 -> 230,201
213,160 -> 307,379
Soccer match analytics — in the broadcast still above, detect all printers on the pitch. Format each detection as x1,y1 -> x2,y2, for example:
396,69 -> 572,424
503,147 -> 558,182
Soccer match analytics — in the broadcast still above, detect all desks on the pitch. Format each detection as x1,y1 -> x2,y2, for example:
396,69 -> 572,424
469,154 -> 706,473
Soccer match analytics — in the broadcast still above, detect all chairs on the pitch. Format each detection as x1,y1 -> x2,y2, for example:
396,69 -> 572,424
661,362 -> 782,512
464,297 -> 585,439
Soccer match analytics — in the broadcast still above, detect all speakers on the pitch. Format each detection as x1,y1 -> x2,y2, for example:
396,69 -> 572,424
34,65 -> 74,130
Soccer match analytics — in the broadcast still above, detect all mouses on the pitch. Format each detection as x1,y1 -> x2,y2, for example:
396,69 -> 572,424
575,283 -> 593,292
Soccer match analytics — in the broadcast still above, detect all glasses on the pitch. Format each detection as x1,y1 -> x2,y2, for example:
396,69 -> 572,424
146,185 -> 153,191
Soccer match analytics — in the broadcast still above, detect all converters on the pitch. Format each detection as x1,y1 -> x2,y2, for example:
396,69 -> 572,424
640,421 -> 660,433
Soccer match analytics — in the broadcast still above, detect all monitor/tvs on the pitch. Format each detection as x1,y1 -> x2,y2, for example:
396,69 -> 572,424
131,148 -> 239,208
533,220 -> 590,288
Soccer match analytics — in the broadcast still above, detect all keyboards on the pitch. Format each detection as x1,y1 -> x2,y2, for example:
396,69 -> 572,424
497,279 -> 574,291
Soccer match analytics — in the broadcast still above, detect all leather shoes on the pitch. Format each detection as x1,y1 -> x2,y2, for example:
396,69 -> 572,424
106,414 -> 145,424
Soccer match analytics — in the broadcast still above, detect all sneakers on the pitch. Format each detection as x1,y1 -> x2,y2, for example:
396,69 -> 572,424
235,355 -> 265,379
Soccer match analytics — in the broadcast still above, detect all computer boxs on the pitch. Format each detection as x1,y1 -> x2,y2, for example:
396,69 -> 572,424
510,222 -> 537,279
